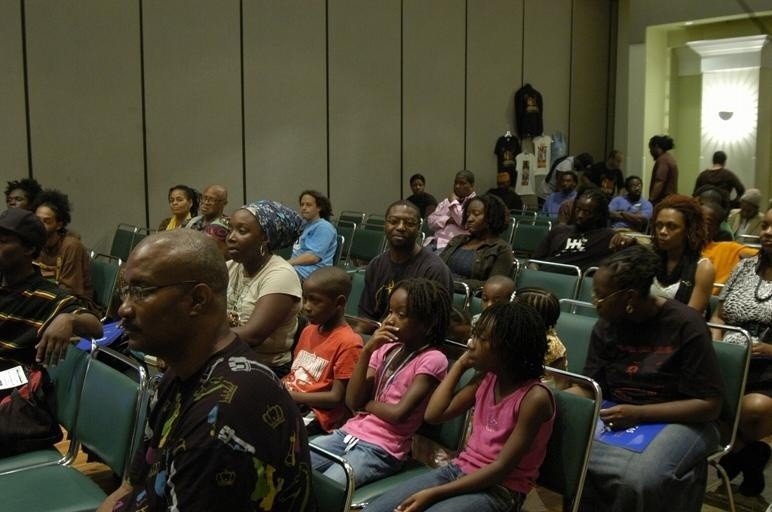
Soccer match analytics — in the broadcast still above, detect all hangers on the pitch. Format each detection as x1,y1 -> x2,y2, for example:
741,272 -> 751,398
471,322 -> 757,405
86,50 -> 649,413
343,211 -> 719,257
494,126 -> 550,157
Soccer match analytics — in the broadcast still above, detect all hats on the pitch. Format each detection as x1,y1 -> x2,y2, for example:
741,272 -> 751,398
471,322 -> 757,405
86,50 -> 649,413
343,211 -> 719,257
739,188 -> 761,205
0,207 -> 47,248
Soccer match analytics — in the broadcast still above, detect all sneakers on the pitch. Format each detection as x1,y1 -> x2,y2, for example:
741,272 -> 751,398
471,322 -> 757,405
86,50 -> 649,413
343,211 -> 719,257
717,440 -> 771,496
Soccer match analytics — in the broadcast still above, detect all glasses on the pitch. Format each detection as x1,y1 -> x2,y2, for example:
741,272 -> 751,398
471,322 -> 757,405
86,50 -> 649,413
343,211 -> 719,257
119,279 -> 197,304
588,288 -> 634,306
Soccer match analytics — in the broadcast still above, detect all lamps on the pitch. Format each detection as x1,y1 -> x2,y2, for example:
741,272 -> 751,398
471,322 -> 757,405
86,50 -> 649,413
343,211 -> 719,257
718,112 -> 733,120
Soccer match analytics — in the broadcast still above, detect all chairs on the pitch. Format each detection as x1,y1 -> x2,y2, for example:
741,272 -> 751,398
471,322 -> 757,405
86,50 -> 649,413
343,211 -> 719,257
0,204 -> 772,512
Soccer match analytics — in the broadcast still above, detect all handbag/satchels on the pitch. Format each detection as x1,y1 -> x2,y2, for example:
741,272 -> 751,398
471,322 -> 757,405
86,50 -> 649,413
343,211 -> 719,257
0,364 -> 63,456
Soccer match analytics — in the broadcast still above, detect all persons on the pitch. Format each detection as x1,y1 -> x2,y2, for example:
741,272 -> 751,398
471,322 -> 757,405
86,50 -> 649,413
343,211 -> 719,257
275,267 -> 571,512
163,184 -> 338,381
1,178 -> 104,399
531,134 -> 771,512
117,229 -> 312,512
351,169 -> 515,346
